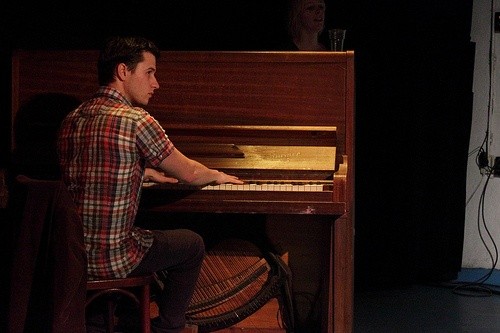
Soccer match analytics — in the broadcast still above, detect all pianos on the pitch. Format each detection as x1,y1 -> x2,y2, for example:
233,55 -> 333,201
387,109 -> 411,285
10,48 -> 354,333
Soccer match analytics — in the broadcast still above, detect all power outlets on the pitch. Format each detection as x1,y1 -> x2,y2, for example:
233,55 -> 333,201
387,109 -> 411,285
480,156 -> 496,174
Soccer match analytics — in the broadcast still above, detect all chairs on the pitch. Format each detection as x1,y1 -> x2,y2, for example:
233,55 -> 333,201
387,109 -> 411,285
0,174 -> 157,333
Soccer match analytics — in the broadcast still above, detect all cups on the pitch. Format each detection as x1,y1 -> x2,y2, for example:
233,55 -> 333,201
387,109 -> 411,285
328,29 -> 346,51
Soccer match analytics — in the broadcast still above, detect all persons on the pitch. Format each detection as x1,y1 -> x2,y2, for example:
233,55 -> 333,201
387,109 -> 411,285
56,36 -> 244,332
285,1 -> 327,50
